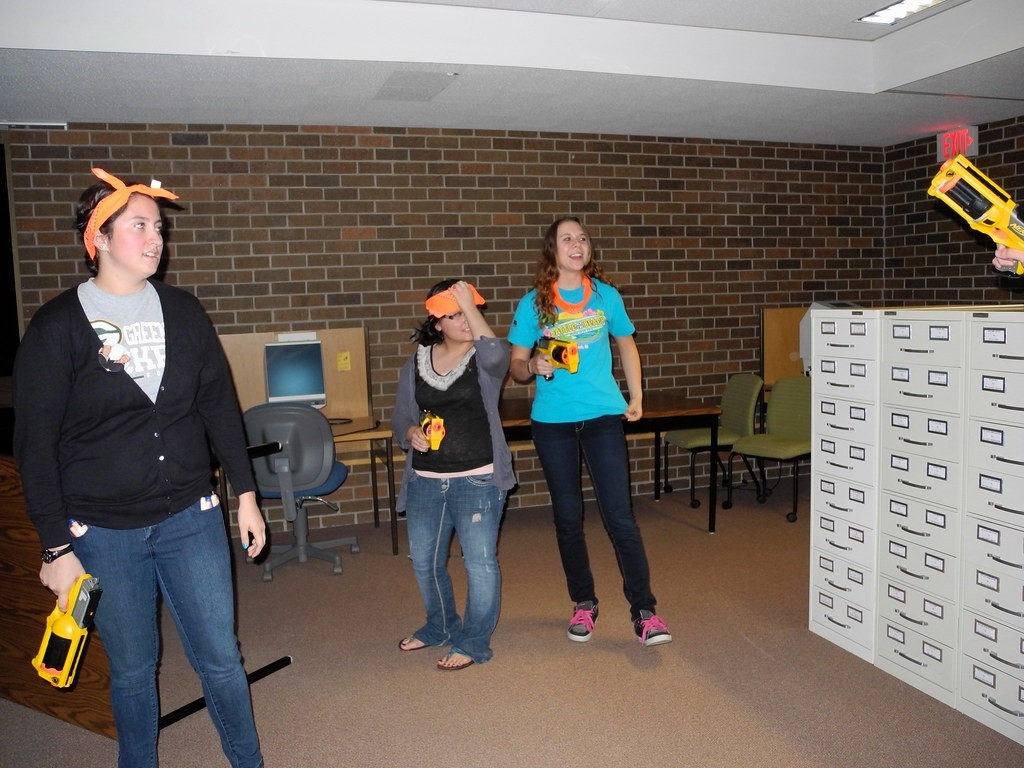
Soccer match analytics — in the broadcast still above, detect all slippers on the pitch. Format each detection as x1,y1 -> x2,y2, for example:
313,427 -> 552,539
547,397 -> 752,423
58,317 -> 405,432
398,634 -> 430,651
437,650 -> 474,670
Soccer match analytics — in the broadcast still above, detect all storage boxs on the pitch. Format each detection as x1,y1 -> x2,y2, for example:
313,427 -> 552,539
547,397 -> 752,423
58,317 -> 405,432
217,326 -> 377,436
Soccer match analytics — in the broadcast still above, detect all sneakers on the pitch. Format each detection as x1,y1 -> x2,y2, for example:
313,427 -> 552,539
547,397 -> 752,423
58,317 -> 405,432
567,601 -> 598,642
630,609 -> 671,647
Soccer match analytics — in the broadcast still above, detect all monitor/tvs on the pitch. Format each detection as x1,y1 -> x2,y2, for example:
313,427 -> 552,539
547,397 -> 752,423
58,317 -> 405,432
264,341 -> 327,409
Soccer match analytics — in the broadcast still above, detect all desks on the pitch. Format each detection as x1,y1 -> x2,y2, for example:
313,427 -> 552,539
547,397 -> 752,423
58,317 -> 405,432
496,388 -> 721,536
213,423 -> 398,556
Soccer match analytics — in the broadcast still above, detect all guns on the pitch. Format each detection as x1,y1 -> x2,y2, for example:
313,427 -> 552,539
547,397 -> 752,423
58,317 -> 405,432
925,151 -> 1024,280
31,573 -> 103,692
420,409 -> 448,452
540,336 -> 581,386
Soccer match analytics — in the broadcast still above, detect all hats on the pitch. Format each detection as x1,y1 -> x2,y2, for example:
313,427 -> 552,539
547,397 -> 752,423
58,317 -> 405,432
424,282 -> 487,318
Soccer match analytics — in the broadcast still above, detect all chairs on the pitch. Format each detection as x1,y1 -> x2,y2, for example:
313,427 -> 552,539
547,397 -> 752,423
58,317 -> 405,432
241,403 -> 360,583
662,372 -> 765,509
721,375 -> 811,521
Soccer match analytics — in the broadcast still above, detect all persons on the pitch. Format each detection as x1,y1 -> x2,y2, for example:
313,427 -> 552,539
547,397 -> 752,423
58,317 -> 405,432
507,218 -> 672,649
390,278 -> 518,669
5,176 -> 269,758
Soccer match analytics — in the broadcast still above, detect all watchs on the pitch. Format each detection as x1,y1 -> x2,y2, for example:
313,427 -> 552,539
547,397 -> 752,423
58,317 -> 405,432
40,545 -> 74,564
527,358 -> 533,375
